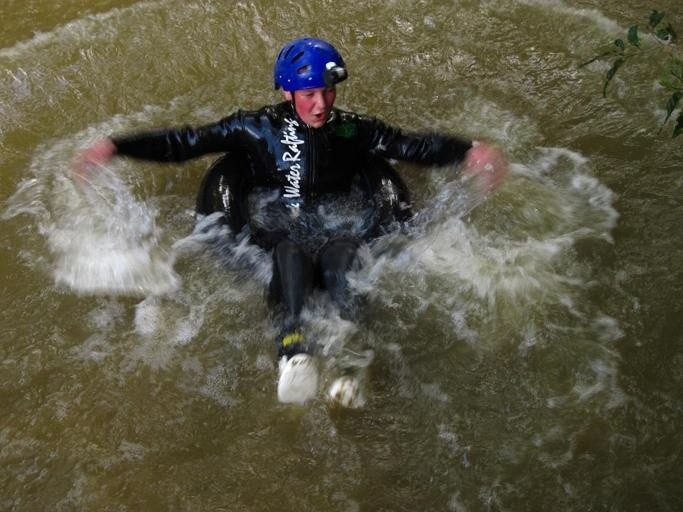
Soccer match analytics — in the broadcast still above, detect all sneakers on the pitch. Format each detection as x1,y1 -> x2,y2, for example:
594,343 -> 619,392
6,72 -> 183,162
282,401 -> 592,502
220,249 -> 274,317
277,352 -> 319,406
328,376 -> 362,409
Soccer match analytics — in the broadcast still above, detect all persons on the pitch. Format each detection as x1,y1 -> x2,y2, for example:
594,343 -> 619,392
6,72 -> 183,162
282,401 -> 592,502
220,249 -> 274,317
69,38 -> 504,409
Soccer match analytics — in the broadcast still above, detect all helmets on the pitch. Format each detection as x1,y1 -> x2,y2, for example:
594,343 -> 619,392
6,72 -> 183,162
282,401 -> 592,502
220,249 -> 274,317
274,37 -> 348,92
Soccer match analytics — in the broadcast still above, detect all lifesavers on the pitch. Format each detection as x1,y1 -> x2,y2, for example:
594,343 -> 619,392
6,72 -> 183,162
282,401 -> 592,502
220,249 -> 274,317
192,150 -> 411,283
